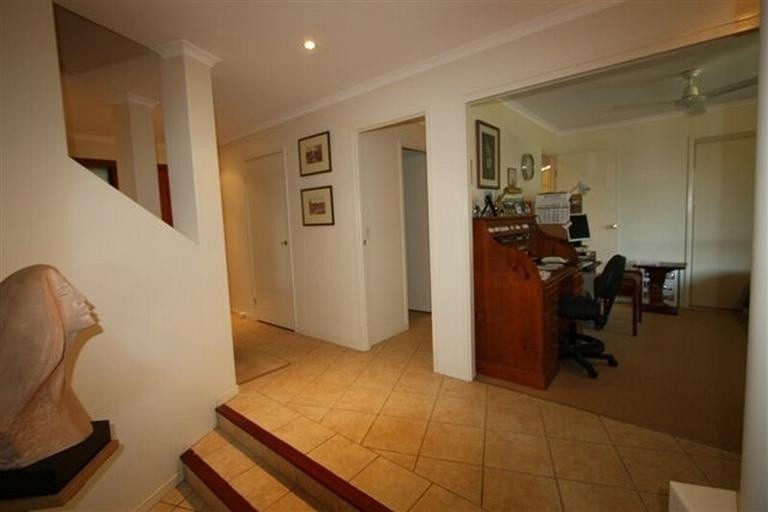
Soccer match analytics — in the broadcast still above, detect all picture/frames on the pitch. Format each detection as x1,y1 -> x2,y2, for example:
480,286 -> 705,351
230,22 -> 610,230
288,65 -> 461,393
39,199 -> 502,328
298,131 -> 332,177
476,120 -> 500,189
301,186 -> 334,226
479,189 -> 530,217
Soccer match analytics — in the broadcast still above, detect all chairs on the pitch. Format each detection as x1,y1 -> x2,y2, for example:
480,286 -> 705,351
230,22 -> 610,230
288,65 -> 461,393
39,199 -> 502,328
558,254 -> 626,379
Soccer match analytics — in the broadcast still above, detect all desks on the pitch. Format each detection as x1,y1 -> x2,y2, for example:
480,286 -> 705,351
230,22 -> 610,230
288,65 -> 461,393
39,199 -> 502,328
473,214 -> 584,390
582,261 -> 602,302
632,261 -> 687,315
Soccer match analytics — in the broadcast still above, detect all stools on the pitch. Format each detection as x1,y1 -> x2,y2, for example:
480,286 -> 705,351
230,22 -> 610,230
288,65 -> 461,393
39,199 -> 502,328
594,270 -> 643,336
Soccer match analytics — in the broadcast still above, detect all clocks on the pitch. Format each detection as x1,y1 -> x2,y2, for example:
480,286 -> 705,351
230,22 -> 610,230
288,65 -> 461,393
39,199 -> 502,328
521,153 -> 534,180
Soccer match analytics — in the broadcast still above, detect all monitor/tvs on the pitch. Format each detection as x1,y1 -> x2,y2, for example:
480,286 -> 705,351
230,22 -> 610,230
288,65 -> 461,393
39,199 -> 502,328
567,213 -> 592,246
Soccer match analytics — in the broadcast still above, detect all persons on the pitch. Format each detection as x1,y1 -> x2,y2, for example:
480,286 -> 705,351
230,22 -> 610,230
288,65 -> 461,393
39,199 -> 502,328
1,262 -> 99,475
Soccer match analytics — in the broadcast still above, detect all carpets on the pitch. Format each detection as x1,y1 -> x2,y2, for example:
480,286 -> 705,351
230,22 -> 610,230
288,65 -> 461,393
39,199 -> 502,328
234,344 -> 291,385
475,303 -> 746,455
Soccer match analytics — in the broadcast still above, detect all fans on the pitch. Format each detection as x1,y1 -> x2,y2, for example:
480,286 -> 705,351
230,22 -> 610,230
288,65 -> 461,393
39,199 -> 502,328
609,68 -> 758,116
